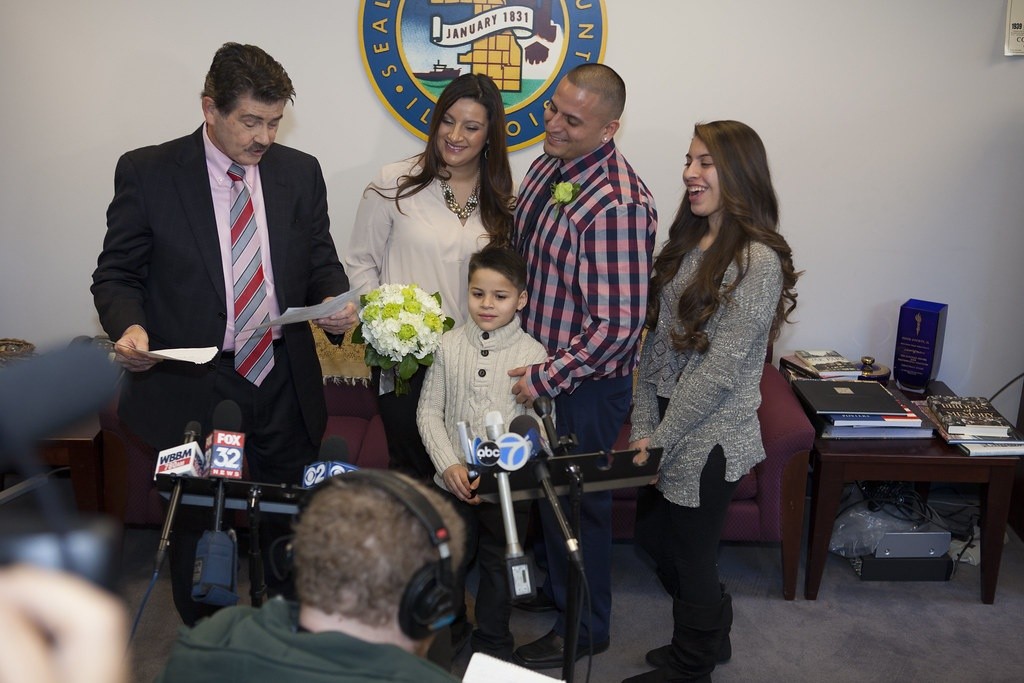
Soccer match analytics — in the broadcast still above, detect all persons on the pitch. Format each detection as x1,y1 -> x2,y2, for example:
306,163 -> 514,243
417,247 -> 547,661
88,44 -> 354,607
622,120 -> 803,683
511,64 -> 657,667
153,469 -> 465,682
347,73 -> 515,488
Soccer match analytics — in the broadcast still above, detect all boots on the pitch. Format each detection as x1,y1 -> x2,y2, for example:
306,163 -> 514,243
621,582 -> 732,683
645,593 -> 732,668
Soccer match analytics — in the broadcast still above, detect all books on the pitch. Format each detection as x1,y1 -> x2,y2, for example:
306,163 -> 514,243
792,379 -> 938,439
916,395 -> 1024,456
779,349 -> 862,379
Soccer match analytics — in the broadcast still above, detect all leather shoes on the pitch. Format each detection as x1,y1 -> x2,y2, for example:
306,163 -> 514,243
511,587 -> 558,614
511,628 -> 610,671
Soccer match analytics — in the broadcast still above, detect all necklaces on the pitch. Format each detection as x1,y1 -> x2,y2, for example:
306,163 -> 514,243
439,168 -> 481,219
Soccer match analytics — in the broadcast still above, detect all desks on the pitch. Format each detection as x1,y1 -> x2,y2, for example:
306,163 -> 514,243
804,380 -> 1020,604
28,411 -> 101,513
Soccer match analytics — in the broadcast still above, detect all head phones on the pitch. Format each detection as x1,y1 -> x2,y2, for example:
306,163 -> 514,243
284,468 -> 463,640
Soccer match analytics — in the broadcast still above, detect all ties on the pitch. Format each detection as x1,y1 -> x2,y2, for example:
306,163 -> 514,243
226,160 -> 276,388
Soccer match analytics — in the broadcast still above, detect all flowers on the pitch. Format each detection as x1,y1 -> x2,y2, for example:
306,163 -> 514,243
550,180 -> 580,208
349,283 -> 456,396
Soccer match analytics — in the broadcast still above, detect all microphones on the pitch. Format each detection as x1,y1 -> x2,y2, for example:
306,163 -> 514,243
483,409 -> 537,605
303,435 -> 361,490
192,399 -> 246,596
509,416 -> 584,571
532,396 -> 562,454
154,422 -> 206,574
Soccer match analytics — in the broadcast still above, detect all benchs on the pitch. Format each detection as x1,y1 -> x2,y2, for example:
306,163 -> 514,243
98,319 -> 815,601
